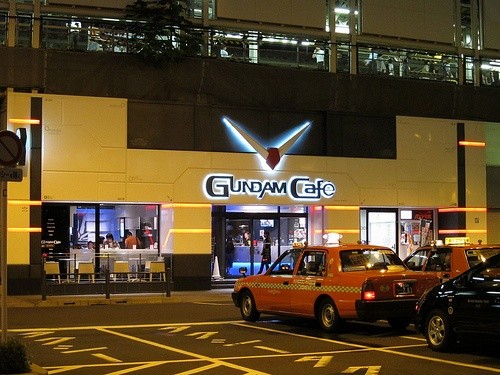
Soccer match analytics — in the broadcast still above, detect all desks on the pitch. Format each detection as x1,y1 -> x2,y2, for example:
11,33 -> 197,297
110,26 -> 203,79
59,259 -> 78,283
129,258 -> 146,283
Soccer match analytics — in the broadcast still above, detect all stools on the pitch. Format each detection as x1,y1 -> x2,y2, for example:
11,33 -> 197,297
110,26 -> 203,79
77,262 -> 95,284
45,261 -> 61,284
114,261 -> 131,282
150,261 -> 166,282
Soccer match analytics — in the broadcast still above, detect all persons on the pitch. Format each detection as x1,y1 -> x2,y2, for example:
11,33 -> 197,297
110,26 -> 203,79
84,241 -> 95,249
257,232 -> 272,273
105,233 -> 120,250
125,230 -> 140,249
224,236 -> 236,274
100,236 -> 105,249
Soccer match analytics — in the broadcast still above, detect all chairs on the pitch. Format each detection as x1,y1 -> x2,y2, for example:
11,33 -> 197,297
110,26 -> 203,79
429,257 -> 446,271
293,260 -> 316,276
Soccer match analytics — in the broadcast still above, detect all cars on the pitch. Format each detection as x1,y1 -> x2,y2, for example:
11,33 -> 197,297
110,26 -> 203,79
404,237 -> 500,299
232,244 -> 442,332
416,254 -> 500,352
